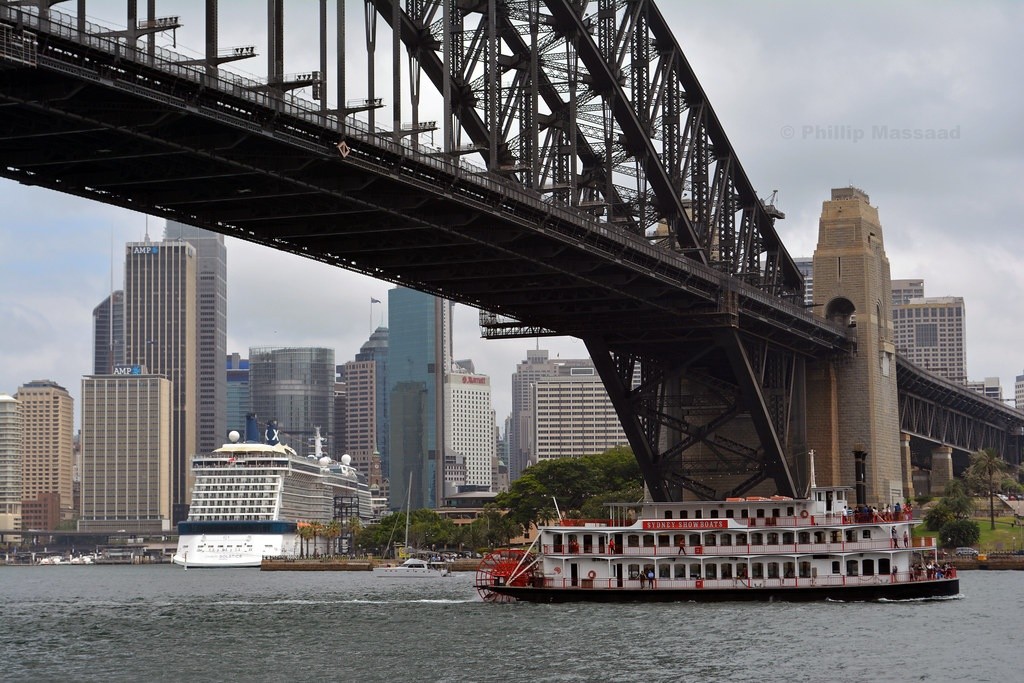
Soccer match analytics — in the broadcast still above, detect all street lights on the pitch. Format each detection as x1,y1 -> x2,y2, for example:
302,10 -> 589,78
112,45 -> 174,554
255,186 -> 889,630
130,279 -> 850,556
458,514 -> 471,533
948,538 -> 953,560
475,515 -> 489,550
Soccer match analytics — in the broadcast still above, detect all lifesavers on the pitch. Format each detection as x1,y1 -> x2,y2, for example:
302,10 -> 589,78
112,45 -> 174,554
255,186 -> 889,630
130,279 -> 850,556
588,570 -> 596,578
800,510 -> 808,518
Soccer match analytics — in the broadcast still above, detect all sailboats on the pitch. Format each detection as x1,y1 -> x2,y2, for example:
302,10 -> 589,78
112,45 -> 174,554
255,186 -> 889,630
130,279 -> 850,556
374,471 -> 441,577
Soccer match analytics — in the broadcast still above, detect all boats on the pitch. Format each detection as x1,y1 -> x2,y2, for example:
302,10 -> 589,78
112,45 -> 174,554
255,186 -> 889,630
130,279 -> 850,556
40,557 -> 60,564
473,451 -> 962,608
174,423 -> 375,568
71,557 -> 91,565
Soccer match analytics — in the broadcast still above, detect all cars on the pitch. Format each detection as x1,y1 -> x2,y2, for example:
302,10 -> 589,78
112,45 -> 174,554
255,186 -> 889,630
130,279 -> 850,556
442,553 -> 461,559
928,550 -> 947,556
464,551 -> 482,558
997,494 -> 1023,500
418,551 -> 445,562
955,548 -> 979,557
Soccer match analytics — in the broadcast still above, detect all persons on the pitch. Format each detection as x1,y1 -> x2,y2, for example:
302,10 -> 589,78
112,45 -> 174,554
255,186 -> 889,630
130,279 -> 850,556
648,570 -> 654,589
411,553 -> 447,562
569,539 -> 579,553
892,526 -> 899,548
678,538 -> 685,554
843,503 -> 900,523
636,571 -> 647,588
892,566 -> 898,583
910,562 -> 954,581
904,531 -> 909,548
610,538 -> 615,554
